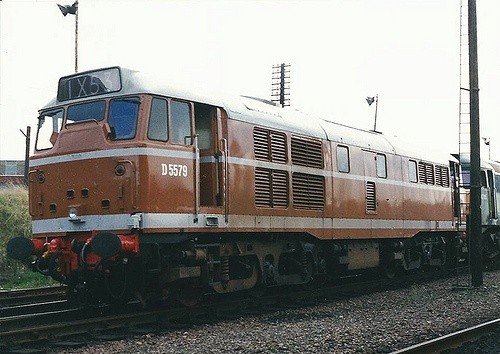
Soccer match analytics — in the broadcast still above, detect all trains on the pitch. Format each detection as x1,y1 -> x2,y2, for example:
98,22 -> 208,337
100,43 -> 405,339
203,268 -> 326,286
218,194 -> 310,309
8,62 -> 500,307
